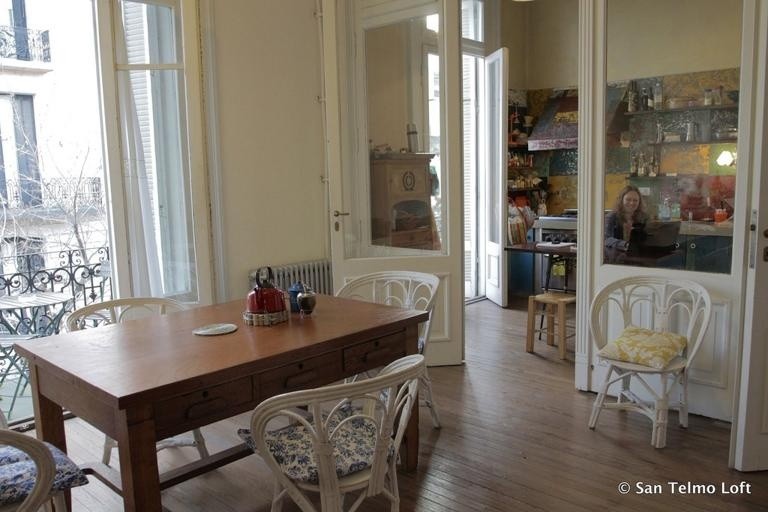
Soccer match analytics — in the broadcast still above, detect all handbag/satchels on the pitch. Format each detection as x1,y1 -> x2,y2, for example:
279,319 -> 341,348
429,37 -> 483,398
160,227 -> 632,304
604,237 -> 630,263
509,216 -> 527,245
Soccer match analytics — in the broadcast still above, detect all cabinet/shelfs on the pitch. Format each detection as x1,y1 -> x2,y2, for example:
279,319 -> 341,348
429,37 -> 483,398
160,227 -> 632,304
508,108 -> 542,194
619,101 -> 738,182
374,152 -> 436,250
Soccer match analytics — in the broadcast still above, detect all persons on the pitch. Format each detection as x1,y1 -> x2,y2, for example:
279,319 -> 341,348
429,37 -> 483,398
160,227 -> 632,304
603,181 -> 652,257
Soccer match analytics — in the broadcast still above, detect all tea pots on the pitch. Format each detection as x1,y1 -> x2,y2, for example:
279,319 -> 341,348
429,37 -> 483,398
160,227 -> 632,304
680,121 -> 697,143
244,266 -> 286,323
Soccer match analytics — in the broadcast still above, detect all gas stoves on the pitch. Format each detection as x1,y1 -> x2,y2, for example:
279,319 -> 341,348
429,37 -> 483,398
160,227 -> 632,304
552,212 -> 577,217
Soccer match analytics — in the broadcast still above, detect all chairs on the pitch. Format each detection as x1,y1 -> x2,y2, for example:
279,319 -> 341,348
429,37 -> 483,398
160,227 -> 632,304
586,274 -> 712,450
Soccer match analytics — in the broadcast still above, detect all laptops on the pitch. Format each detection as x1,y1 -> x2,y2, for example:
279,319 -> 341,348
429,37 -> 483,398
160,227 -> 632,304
624,220 -> 681,256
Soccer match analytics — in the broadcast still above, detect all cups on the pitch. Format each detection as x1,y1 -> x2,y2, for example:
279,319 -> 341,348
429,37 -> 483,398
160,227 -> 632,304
406,122 -> 421,153
649,154 -> 661,176
640,154 -> 648,177
629,151 -> 639,175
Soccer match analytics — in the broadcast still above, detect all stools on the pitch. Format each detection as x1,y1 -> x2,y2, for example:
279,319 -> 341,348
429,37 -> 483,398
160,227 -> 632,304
525,293 -> 577,360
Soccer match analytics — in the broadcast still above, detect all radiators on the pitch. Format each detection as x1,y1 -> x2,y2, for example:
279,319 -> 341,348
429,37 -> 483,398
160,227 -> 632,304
247,258 -> 333,297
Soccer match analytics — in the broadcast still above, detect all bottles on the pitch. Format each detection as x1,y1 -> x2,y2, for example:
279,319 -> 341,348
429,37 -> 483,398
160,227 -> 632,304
641,79 -> 712,109
662,198 -> 672,221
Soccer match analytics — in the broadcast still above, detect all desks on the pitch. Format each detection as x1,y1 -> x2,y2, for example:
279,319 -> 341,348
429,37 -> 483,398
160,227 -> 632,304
503,242 -> 578,341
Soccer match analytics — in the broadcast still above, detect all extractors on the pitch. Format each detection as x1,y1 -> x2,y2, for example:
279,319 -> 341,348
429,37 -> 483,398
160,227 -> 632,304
528,88 -> 630,152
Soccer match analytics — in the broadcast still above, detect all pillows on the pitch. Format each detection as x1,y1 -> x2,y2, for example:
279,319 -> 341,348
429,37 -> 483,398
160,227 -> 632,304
594,325 -> 689,371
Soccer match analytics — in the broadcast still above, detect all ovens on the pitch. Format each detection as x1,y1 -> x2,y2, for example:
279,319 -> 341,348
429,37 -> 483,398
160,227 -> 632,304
541,229 -> 576,293
